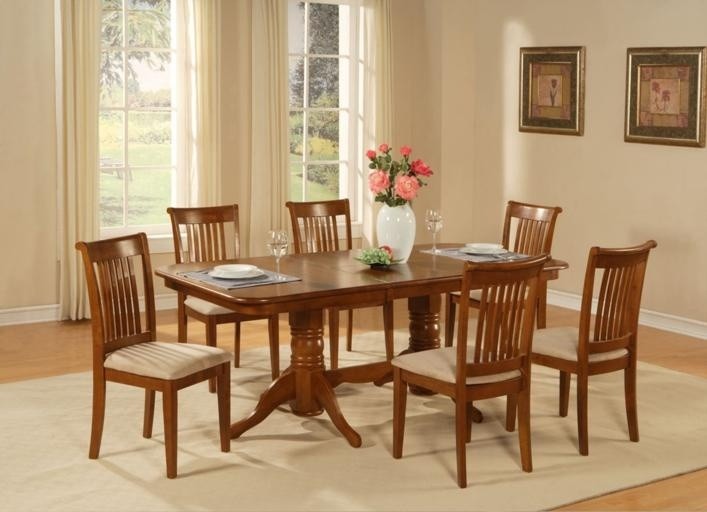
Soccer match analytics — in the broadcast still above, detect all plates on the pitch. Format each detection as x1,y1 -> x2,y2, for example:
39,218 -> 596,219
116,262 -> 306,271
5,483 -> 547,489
214,263 -> 256,275
459,247 -> 508,256
466,243 -> 503,253
207,270 -> 264,279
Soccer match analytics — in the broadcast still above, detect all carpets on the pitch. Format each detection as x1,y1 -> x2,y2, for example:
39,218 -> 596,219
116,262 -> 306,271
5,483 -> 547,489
0,319 -> 707,512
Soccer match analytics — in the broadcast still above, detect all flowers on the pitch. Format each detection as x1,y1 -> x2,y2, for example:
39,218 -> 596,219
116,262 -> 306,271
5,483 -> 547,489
367,144 -> 433,207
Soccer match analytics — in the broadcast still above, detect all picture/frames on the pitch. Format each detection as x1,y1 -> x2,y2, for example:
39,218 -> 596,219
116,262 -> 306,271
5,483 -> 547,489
624,46 -> 707,149
519,46 -> 585,135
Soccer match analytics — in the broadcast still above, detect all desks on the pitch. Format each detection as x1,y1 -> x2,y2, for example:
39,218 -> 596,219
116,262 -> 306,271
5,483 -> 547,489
155,242 -> 569,448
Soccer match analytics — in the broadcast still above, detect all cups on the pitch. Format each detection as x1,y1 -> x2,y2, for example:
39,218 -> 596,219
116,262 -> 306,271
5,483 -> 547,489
424,208 -> 444,255
266,230 -> 290,281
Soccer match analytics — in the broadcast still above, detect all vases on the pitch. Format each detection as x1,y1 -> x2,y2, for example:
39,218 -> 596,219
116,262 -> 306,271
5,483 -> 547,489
377,202 -> 417,263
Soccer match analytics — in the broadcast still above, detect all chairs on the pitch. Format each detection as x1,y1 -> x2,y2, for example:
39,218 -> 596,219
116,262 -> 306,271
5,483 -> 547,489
74,233 -> 233,479
167,204 -> 280,392
444,200 -> 562,346
505,239 -> 659,456
285,198 -> 396,368
390,255 -> 554,489
